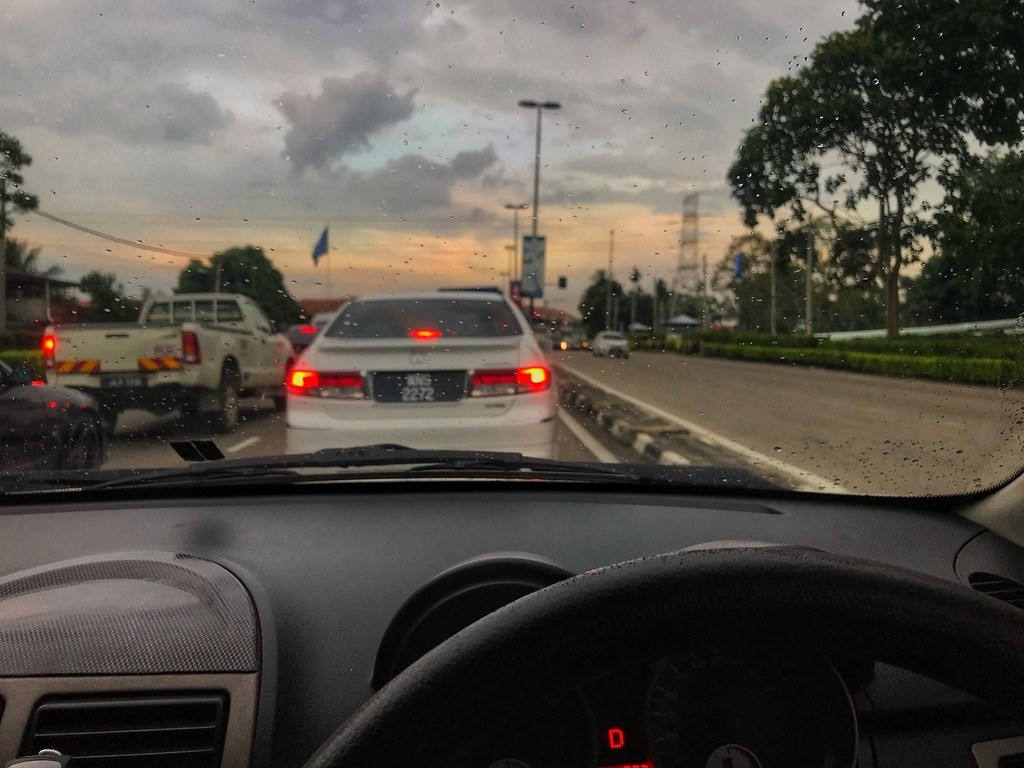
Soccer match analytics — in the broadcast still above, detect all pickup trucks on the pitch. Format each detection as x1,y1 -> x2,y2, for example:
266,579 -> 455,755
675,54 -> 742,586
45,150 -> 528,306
46,292 -> 298,436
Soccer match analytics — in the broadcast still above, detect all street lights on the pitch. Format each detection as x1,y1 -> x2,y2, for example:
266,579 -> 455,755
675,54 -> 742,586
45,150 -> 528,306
506,203 -> 528,303
516,100 -> 563,326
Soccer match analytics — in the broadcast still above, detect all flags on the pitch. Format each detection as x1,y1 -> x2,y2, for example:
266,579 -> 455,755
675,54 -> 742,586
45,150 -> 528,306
313,226 -> 328,266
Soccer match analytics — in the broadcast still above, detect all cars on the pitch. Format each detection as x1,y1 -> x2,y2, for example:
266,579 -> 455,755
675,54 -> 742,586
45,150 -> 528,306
0,355 -> 107,476
288,292 -> 560,467
593,331 -> 630,359
